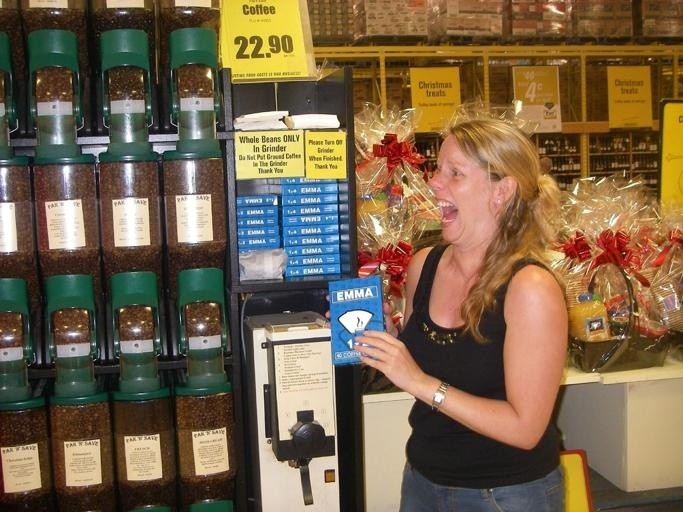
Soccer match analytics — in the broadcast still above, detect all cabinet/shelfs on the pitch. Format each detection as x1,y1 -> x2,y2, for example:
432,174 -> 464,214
313,44 -> 683,208
1,68 -> 364,512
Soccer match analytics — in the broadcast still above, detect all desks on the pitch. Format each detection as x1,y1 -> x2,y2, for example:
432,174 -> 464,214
558,356 -> 683,494
362,357 -> 603,512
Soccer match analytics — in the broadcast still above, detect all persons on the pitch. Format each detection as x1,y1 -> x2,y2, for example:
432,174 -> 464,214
325,119 -> 569,512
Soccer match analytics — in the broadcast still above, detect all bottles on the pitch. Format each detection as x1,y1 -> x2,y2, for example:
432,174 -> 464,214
569,293 -> 609,342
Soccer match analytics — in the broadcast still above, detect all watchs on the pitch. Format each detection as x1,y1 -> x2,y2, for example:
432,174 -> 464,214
432,382 -> 449,411
